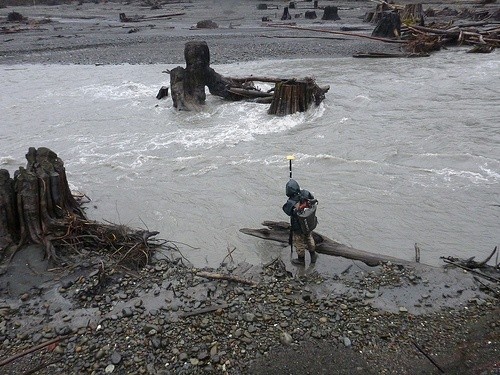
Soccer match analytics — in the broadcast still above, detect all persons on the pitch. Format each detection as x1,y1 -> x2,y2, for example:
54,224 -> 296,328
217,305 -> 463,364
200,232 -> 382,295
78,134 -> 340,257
281,179 -> 320,266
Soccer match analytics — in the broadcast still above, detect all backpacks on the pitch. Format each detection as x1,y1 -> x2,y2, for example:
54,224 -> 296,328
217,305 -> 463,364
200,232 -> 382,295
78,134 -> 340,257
291,196 -> 318,236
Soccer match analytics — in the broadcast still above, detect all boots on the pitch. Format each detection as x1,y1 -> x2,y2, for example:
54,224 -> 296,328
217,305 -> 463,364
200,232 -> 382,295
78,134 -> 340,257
291,256 -> 305,265
310,251 -> 316,263
